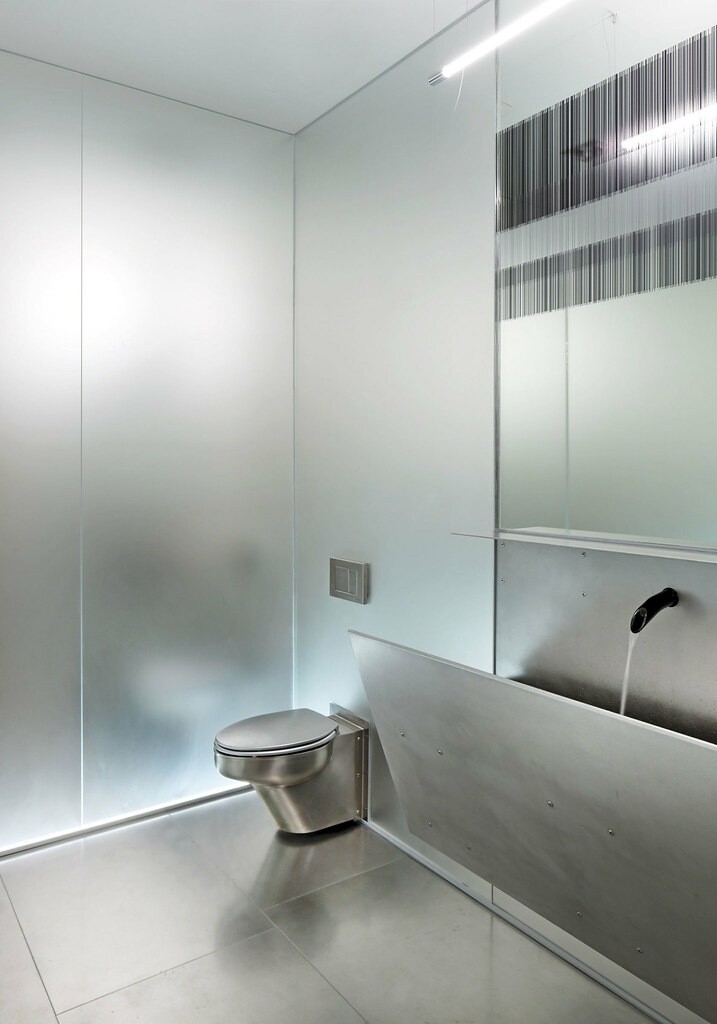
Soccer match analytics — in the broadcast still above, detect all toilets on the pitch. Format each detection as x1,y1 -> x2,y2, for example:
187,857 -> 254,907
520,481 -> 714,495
213,703 -> 370,838
